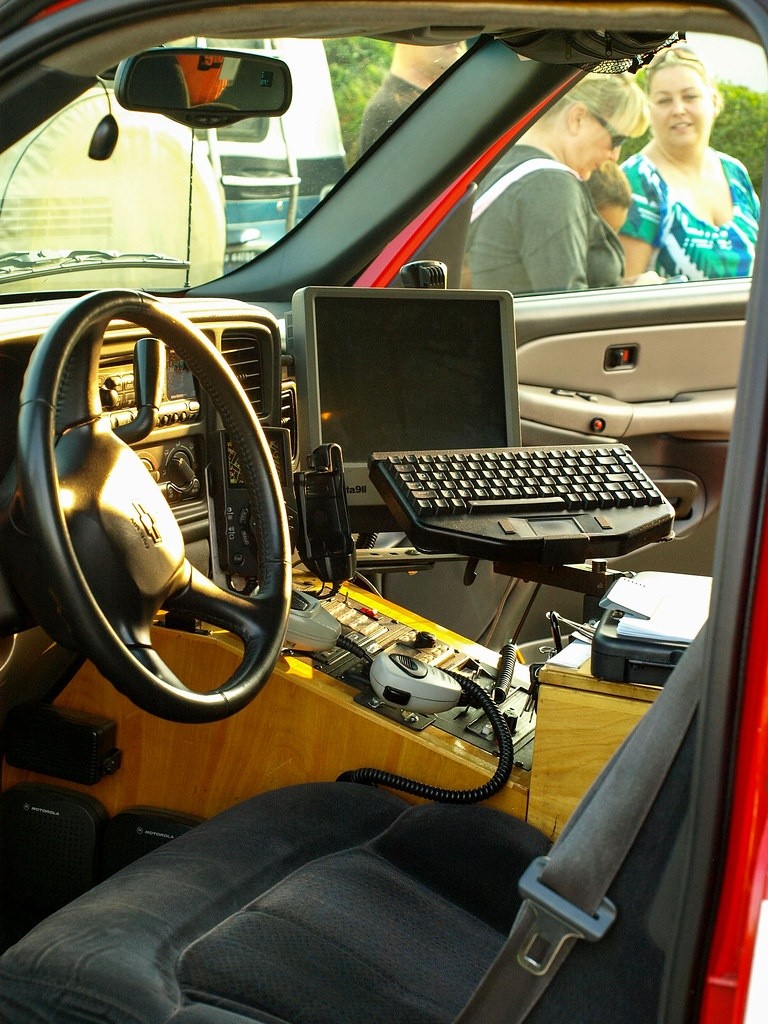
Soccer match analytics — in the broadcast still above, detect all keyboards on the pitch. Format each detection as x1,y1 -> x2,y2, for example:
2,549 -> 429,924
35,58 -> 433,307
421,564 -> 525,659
367,444 -> 676,563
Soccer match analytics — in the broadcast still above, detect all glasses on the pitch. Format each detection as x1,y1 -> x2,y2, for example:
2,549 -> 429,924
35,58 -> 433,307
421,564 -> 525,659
587,111 -> 628,150
644,47 -> 705,72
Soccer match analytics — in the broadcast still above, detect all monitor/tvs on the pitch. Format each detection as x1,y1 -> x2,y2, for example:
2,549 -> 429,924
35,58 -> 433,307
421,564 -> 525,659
292,285 -> 521,506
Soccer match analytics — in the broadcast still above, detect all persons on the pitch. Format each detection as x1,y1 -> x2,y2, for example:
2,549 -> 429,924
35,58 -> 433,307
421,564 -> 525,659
360,39 -> 467,158
616,46 -> 761,284
459,70 -> 666,295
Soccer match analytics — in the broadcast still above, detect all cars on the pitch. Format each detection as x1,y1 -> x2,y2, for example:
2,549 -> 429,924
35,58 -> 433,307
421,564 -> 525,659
0,0 -> 763,1024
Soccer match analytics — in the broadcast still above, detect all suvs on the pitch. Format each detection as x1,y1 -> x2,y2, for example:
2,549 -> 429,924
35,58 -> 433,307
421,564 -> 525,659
1,36 -> 348,292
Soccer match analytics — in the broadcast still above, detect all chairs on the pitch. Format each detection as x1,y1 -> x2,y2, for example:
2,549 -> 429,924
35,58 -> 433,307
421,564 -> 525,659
0,618 -> 727,1024
